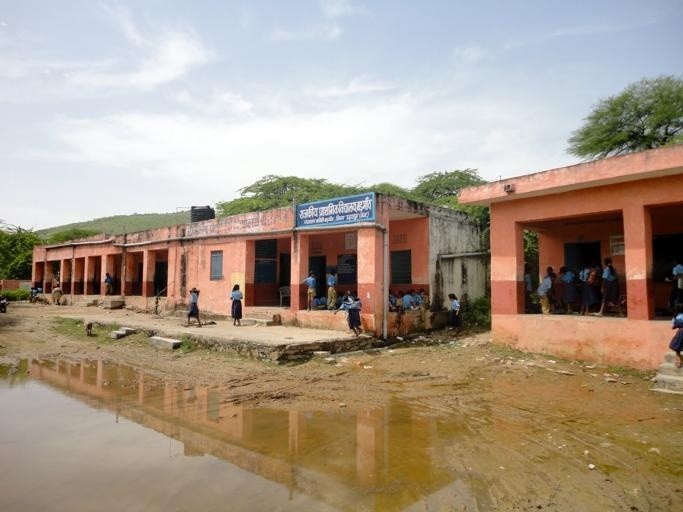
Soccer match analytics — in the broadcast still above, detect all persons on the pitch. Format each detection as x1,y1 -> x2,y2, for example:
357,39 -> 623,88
302,266 -> 424,338
104,272 -> 112,294
670,259 -> 683,314
669,303 -> 683,368
525,258 -> 626,314
229,284 -> 242,326
184,288 -> 201,328
448,293 -> 460,331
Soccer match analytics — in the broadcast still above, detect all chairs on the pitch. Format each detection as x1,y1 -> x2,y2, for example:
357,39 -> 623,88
278,285 -> 292,307
